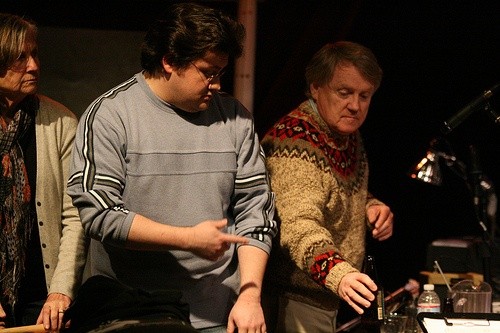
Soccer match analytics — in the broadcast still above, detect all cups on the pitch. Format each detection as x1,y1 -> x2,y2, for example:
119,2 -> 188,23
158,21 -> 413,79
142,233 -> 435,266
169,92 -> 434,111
380,315 -> 408,333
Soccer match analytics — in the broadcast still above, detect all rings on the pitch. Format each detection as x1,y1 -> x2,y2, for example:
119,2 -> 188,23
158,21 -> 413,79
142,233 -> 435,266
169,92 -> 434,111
58,310 -> 65,312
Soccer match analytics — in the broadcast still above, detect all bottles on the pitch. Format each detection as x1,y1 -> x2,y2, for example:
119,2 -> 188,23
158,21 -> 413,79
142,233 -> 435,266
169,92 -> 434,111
361,255 -> 384,327
415,284 -> 441,333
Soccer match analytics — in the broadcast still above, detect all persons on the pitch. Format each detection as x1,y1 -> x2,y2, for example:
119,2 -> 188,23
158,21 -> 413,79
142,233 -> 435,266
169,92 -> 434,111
258,42 -> 393,333
0,12 -> 91,333
66,2 -> 279,333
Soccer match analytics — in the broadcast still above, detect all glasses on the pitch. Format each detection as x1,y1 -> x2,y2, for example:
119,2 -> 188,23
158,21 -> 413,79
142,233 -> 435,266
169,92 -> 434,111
189,60 -> 226,81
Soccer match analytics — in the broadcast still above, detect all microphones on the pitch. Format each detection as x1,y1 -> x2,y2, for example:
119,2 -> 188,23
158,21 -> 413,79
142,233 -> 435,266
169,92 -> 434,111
439,82 -> 500,135
